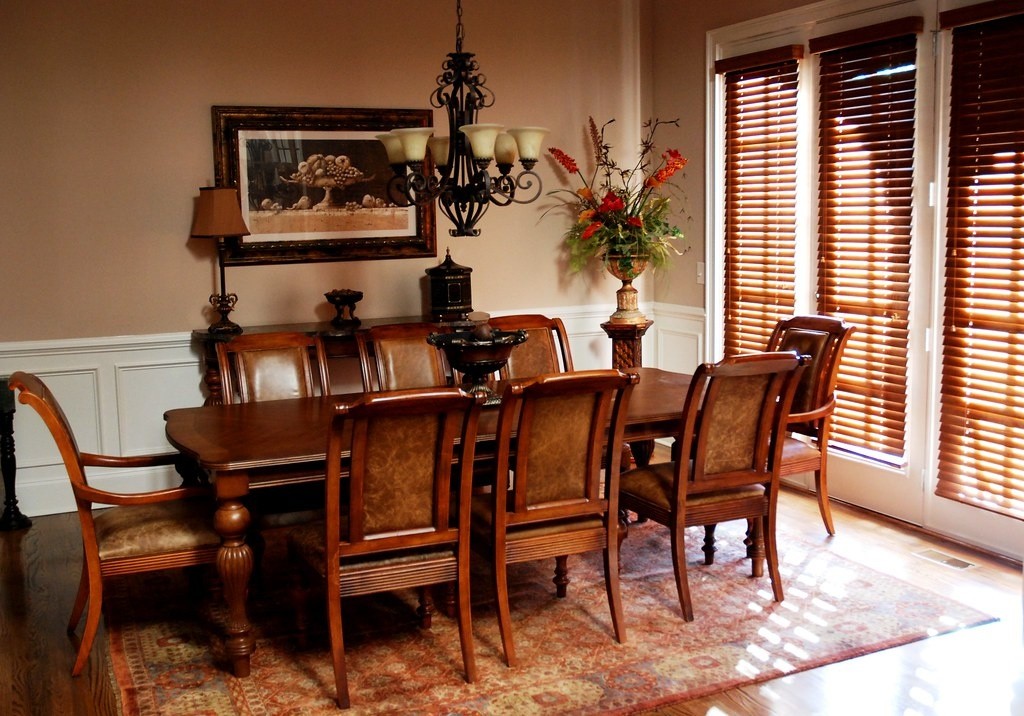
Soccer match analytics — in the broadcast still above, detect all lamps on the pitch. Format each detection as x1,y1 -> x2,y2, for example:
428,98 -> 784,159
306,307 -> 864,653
190,186 -> 251,335
375,0 -> 551,237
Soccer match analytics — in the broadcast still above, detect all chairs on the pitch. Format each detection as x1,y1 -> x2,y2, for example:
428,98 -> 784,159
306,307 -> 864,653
7,372 -> 220,678
615,352 -> 804,622
354,321 -> 448,393
740,316 -> 859,558
216,333 -> 332,405
449,370 -> 640,669
280,386 -> 489,712
489,313 -> 631,524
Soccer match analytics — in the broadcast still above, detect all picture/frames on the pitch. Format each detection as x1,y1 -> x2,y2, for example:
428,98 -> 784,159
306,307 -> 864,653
209,104 -> 438,267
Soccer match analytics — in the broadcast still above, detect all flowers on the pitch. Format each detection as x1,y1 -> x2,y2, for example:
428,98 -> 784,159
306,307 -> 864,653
535,115 -> 695,278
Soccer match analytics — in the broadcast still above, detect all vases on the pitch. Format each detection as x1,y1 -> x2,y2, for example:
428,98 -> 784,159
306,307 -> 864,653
598,254 -> 652,324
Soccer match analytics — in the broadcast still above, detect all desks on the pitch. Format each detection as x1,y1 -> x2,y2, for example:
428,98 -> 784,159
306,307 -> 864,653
190,310 -> 492,395
165,369 -> 765,678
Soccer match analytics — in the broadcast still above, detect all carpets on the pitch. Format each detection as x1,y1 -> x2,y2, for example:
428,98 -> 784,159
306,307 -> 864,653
106,483 -> 998,716
